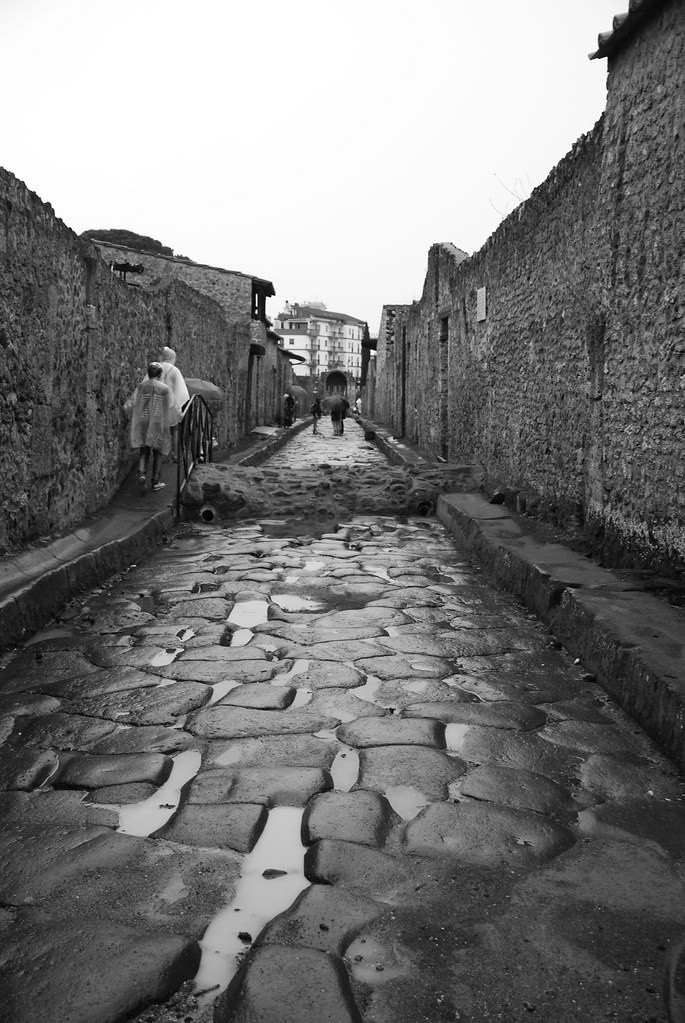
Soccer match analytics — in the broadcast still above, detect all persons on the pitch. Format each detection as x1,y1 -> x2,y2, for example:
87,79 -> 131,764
179,402 -> 207,463
330,409 -> 347,435
311,397 -> 323,435
125,345 -> 190,462
281,393 -> 298,428
130,361 -> 182,493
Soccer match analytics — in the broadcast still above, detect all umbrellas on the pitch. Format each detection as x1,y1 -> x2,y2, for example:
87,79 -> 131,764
322,395 -> 350,411
185,377 -> 226,401
288,386 -> 308,399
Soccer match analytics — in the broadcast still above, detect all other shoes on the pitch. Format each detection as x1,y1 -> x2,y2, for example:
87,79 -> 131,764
173,455 -> 182,463
138,473 -> 147,494
152,481 -> 166,490
